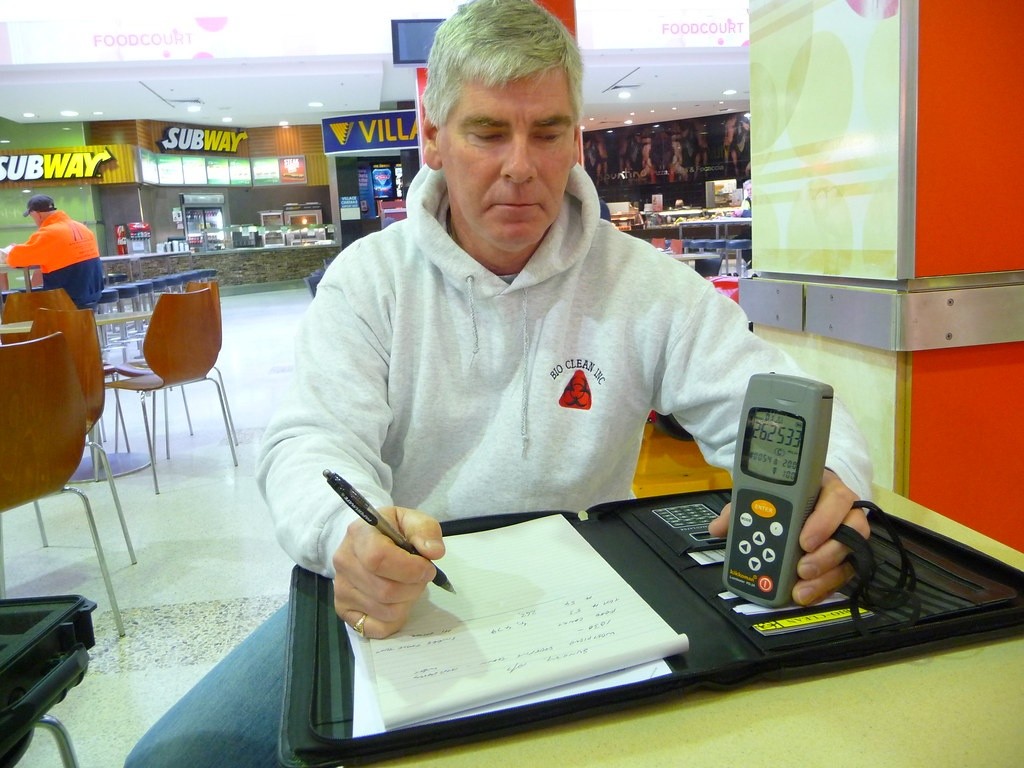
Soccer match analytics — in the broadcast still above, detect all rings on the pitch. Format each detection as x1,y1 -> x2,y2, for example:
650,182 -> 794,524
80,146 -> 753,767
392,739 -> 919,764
353,613 -> 368,637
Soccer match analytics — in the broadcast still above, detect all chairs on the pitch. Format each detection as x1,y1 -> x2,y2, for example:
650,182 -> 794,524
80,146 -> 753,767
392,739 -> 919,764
670,239 -> 683,255
98,289 -> 237,494
111,281 -> 238,459
0,305 -> 137,566
651,238 -> 666,249
0,331 -> 127,637
0,287 -> 107,444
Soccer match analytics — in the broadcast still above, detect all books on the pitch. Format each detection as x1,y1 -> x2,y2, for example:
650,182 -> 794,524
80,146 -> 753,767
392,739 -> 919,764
362,514 -> 690,731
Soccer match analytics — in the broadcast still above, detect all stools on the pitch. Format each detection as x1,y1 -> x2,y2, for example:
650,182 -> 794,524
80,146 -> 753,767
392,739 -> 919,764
0,268 -> 219,365
679,238 -> 692,254
726,239 -> 753,276
690,239 -> 714,253
705,239 -> 727,276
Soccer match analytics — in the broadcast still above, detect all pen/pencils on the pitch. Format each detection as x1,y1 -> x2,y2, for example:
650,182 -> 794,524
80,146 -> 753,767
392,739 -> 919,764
322,469 -> 457,594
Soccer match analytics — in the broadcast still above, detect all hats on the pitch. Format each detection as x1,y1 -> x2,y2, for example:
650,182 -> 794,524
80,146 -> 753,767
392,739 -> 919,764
23,194 -> 57,217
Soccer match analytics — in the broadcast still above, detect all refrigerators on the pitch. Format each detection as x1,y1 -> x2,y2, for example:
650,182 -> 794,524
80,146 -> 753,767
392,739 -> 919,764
179,193 -> 227,251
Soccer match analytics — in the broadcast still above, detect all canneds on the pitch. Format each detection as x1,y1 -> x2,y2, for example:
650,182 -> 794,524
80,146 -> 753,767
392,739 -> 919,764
205,211 -> 217,216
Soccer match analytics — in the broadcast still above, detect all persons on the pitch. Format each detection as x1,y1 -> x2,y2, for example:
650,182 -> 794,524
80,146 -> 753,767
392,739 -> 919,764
731,181 -> 752,276
674,200 -> 684,209
6,194 -> 104,311
584,113 -> 751,183
124,0 -> 872,768
599,198 -> 611,221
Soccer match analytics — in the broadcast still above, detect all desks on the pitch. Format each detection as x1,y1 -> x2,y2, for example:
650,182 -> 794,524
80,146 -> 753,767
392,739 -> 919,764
355,482 -> 1024,768
663,249 -> 721,270
0,311 -> 155,485
679,217 -> 753,253
0,250 -> 193,345
